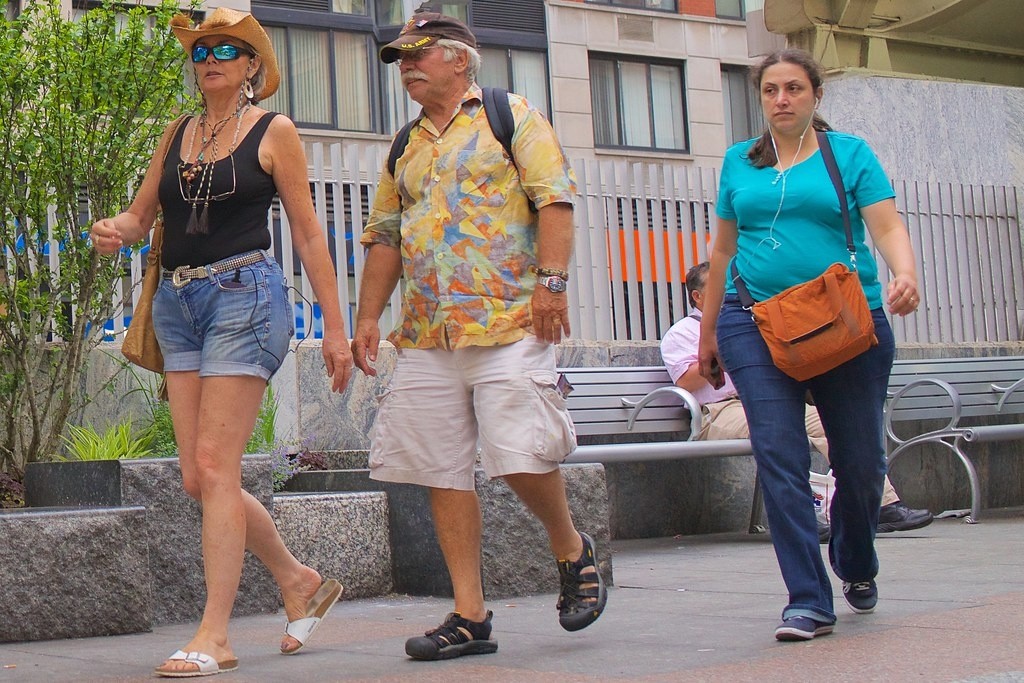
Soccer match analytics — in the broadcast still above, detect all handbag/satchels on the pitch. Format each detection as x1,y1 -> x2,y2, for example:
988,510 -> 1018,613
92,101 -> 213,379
810,470 -> 837,524
120,212 -> 168,403
743,262 -> 877,381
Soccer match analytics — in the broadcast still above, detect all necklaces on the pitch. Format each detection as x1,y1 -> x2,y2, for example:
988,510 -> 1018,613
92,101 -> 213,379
177,100 -> 251,234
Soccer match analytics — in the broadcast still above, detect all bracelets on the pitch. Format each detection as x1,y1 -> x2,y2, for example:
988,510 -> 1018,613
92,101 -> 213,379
535,267 -> 569,281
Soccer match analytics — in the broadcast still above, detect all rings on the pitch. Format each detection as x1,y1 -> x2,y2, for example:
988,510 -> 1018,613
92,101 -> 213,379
553,319 -> 561,329
910,297 -> 915,303
95,235 -> 99,245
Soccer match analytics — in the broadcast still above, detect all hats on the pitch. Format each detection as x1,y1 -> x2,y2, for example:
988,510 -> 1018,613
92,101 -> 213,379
170,6 -> 281,101
379,12 -> 478,64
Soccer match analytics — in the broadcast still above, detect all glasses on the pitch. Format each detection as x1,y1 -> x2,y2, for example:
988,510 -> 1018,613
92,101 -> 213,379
190,45 -> 258,64
400,45 -> 442,60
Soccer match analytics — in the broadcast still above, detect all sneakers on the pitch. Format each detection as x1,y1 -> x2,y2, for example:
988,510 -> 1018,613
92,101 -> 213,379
842,580 -> 878,614
818,522 -> 831,541
876,503 -> 933,533
776,617 -> 834,641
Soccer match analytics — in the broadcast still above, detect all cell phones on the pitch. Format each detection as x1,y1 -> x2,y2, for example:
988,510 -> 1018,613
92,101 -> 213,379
224,281 -> 247,288
710,359 -> 725,389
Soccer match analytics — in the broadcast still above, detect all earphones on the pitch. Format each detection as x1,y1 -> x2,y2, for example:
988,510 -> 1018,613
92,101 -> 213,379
813,97 -> 819,109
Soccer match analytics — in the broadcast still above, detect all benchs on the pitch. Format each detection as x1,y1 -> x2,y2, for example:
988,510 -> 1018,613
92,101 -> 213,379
473,366 -> 764,534
880,356 -> 1024,523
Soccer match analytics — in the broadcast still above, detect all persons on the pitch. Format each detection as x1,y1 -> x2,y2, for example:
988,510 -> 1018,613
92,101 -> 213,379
352,10 -> 607,660
698,51 -> 920,641
660,261 -> 934,546
87,4 -> 354,678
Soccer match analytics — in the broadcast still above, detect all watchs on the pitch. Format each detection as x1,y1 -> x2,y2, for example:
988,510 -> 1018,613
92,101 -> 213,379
537,276 -> 566,293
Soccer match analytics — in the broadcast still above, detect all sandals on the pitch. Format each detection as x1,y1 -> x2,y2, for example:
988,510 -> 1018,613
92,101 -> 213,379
555,532 -> 607,632
405,610 -> 498,662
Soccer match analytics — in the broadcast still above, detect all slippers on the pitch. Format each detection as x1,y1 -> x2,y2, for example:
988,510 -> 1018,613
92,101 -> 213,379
280,579 -> 342,655
155,648 -> 239,677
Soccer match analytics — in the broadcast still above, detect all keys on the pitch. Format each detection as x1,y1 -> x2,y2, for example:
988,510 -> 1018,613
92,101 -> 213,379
185,183 -> 191,203
